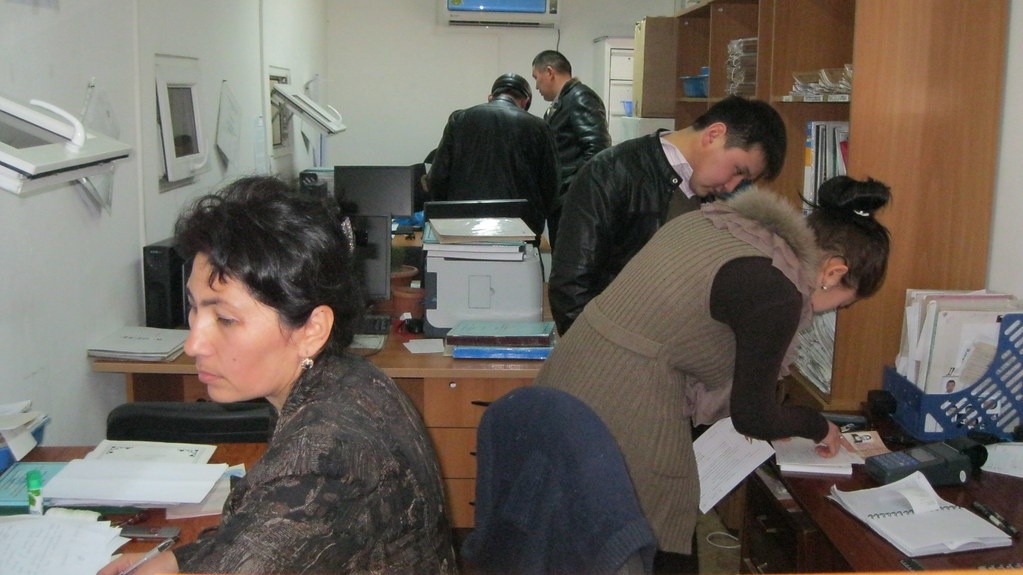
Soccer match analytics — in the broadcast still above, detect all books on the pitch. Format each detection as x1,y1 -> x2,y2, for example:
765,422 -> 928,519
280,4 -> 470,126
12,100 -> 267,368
780,465 -> 1012,557
801,120 -> 849,207
421,217 -> 556,361
88,323 -> 189,361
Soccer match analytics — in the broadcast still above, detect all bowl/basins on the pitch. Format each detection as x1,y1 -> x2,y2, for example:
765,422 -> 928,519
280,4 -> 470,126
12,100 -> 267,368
680,75 -> 708,97
621,100 -> 633,117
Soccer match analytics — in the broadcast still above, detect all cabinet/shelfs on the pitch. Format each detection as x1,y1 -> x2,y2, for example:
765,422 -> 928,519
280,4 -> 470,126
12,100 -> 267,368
675,0 -> 774,131
633,15 -> 677,118
592,36 -> 635,131
770,0 -> 1010,539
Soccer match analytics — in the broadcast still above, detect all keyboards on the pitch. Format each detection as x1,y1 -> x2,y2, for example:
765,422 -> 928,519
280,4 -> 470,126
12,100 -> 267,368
356,315 -> 391,334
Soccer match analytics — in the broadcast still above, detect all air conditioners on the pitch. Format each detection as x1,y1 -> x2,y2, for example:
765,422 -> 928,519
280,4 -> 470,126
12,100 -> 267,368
448,0 -> 562,32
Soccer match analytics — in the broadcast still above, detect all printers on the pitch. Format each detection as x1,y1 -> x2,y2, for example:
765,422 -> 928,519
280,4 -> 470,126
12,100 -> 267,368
419,199 -> 544,338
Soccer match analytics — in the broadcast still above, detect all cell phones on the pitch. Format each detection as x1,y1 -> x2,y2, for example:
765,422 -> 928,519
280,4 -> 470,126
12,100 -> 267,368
119,525 -> 180,541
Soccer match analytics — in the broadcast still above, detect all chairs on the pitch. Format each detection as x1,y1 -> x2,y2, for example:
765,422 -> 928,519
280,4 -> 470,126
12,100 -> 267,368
106,402 -> 278,444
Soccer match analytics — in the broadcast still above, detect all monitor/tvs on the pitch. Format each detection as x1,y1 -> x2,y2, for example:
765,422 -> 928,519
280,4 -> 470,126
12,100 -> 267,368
352,211 -> 394,300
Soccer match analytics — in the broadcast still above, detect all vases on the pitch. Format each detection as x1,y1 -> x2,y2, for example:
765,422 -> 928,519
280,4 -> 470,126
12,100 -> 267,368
390,285 -> 425,318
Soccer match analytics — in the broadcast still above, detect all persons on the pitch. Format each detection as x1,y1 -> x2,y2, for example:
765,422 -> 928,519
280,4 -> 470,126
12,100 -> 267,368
425,74 -> 562,281
946,399 -> 997,430
945,380 -> 956,393
530,50 -> 613,254
547,95 -> 788,341
95,173 -> 459,575
536,175 -> 892,575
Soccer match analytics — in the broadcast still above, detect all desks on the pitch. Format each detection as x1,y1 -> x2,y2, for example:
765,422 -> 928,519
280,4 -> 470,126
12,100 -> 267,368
0,441 -> 269,575
97,282 -> 564,529
739,411 -> 1023,575
391,230 -> 552,249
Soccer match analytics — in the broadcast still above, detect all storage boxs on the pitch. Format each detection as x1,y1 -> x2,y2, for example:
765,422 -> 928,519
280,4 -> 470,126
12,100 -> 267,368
609,115 -> 676,148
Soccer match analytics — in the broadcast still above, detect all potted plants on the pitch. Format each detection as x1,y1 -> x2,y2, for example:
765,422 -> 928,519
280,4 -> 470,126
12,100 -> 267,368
391,245 -> 419,290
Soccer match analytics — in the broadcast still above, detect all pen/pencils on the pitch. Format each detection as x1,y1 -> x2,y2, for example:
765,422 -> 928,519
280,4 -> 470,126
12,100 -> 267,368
119,534 -> 179,575
972,500 -> 1021,539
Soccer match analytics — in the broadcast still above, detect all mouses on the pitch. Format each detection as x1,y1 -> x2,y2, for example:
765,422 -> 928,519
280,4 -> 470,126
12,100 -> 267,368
406,318 -> 424,333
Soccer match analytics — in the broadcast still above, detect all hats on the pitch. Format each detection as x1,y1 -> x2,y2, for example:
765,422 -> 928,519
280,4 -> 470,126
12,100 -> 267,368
492,73 -> 532,111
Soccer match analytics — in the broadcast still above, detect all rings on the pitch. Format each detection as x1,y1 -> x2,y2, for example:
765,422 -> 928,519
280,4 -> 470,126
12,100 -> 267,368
839,435 -> 844,439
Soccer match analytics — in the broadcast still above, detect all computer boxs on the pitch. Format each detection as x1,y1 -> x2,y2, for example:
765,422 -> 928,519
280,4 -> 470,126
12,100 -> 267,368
334,163 -> 426,218
141,235 -> 196,329
